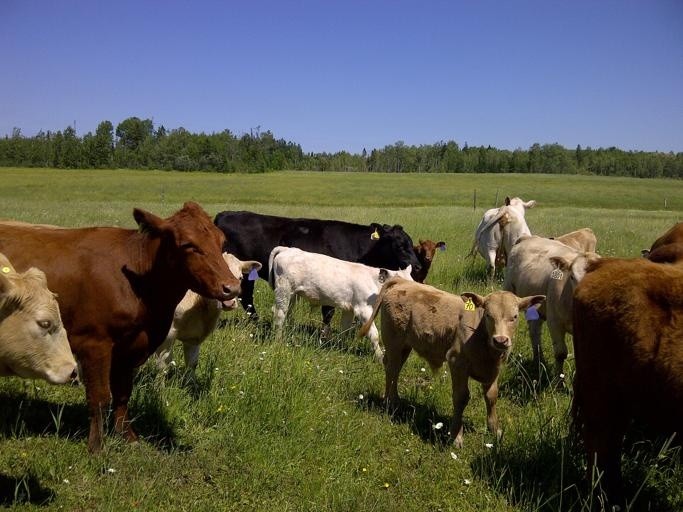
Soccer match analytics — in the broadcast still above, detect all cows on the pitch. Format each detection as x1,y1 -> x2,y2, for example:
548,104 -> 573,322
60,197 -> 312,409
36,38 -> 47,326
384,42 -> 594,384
212,209 -> 446,363
2,220 -> 262,374
3,200 -> 242,452
359,276 -> 546,451
570,220 -> 679,510
463,196 -> 537,287
548,228 -> 597,254
504,232 -> 603,392
1,254 -> 78,386
